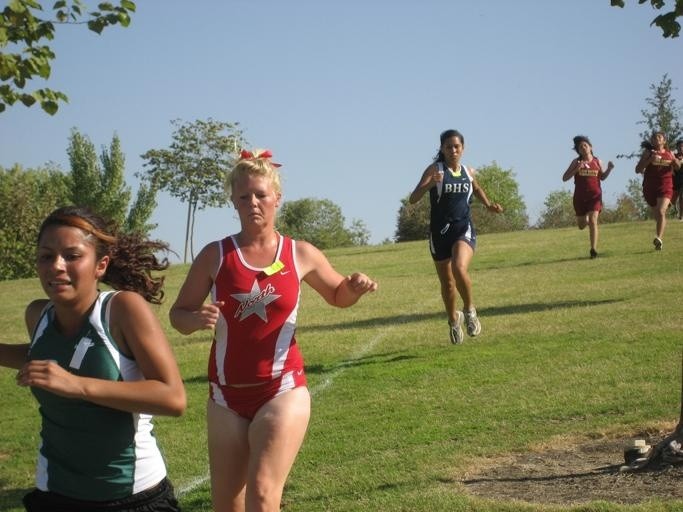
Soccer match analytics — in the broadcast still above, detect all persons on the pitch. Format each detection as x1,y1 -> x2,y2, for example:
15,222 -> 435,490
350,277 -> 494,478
410,130 -> 503,346
0,206 -> 187,511
672,141 -> 683,220
635,133 -> 681,251
169,149 -> 378,512
563,135 -> 615,258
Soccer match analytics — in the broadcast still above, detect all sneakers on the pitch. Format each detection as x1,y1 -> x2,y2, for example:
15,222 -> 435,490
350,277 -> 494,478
448,311 -> 464,344
654,238 -> 662,250
463,308 -> 481,336
